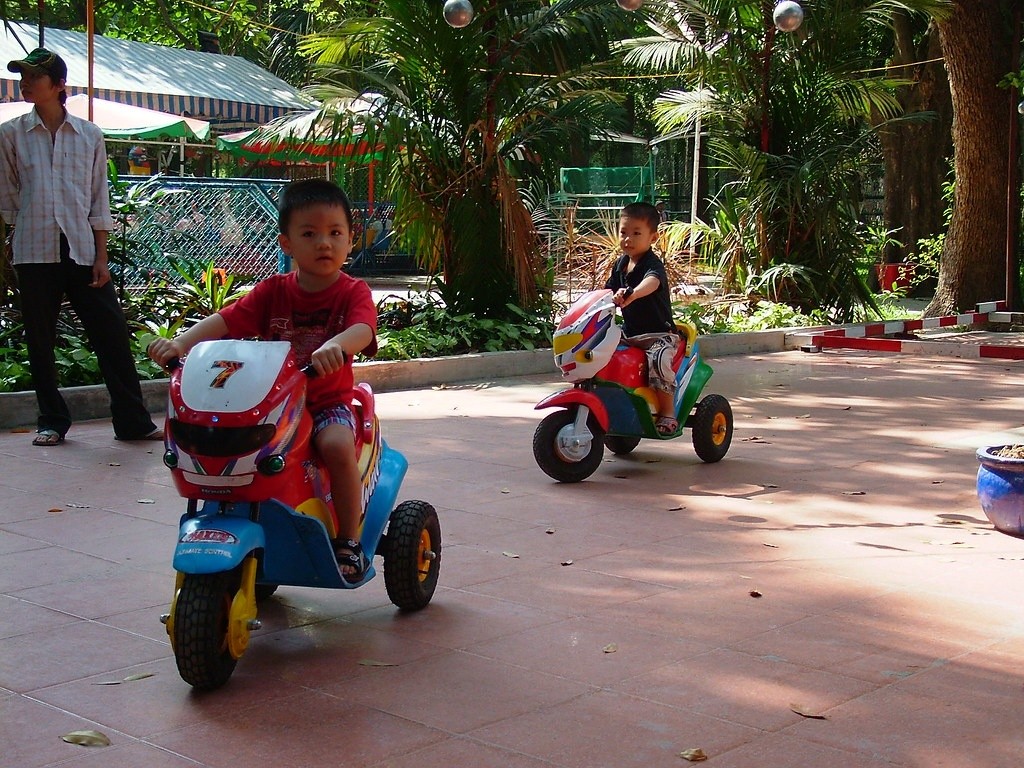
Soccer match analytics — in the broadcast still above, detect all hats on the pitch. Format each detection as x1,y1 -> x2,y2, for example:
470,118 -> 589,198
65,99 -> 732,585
7,48 -> 67,80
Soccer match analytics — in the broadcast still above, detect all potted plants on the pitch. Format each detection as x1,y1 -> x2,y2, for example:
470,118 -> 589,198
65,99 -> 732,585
974,443 -> 1024,535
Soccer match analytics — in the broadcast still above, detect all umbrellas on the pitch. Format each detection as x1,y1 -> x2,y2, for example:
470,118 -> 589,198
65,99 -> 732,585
214,103 -> 413,182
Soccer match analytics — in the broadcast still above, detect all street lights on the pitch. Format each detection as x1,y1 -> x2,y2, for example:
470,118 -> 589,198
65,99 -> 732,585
751,1 -> 805,280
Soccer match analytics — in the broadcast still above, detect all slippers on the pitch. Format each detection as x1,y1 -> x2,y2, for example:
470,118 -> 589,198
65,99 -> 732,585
654,416 -> 679,435
114,427 -> 165,441
32,428 -> 65,446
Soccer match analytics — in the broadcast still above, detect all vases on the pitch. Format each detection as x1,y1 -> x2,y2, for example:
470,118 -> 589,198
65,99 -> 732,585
873,263 -> 918,298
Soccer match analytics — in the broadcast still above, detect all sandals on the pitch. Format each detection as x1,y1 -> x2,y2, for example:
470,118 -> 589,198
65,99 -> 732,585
332,536 -> 371,585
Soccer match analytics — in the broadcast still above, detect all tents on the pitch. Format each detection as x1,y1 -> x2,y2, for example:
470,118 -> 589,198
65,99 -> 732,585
0,20 -> 331,176
1,93 -> 211,143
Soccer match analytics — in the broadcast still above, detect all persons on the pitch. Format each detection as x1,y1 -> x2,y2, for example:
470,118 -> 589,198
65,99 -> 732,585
148,176 -> 380,582
599,203 -> 683,439
0,47 -> 169,446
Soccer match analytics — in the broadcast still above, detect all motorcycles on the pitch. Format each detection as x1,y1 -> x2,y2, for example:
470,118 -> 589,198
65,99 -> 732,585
532,284 -> 733,483
146,339 -> 442,691
